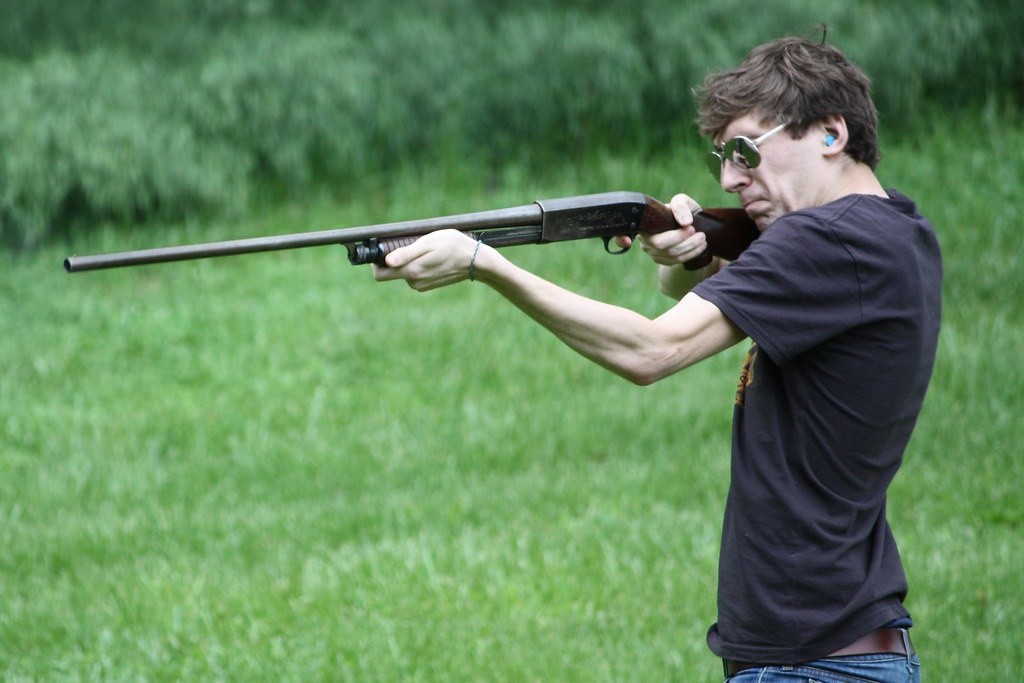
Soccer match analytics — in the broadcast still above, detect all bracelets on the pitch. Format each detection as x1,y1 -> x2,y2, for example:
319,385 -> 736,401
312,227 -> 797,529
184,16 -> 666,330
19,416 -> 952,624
469,240 -> 482,282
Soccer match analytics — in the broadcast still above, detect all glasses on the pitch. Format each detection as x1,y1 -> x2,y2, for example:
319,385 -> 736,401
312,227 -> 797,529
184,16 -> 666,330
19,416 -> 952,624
705,118 -> 801,184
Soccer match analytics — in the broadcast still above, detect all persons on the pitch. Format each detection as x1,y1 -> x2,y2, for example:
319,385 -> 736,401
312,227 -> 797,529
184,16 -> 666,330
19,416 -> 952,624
372,26 -> 944,683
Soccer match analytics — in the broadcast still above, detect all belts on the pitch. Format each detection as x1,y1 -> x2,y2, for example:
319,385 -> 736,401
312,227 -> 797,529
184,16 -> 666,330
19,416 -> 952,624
723,628 -> 916,678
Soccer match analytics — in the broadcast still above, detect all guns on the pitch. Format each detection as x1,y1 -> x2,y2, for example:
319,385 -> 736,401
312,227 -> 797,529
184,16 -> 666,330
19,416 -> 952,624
65,190 -> 758,271
